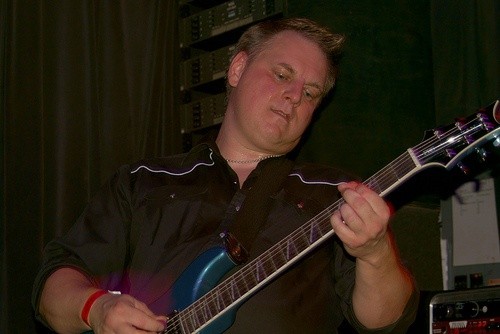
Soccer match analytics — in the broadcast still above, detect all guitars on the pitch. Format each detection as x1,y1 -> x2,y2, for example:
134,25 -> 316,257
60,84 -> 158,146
161,100 -> 500,334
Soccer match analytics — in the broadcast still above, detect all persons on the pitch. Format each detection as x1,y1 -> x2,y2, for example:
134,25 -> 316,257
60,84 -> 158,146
29,17 -> 419,334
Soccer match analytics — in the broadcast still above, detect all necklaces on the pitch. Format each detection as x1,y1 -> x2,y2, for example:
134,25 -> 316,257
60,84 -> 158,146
225,155 -> 280,164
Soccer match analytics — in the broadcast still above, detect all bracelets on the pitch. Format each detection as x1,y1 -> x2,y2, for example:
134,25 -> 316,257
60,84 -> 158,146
81,289 -> 109,328
